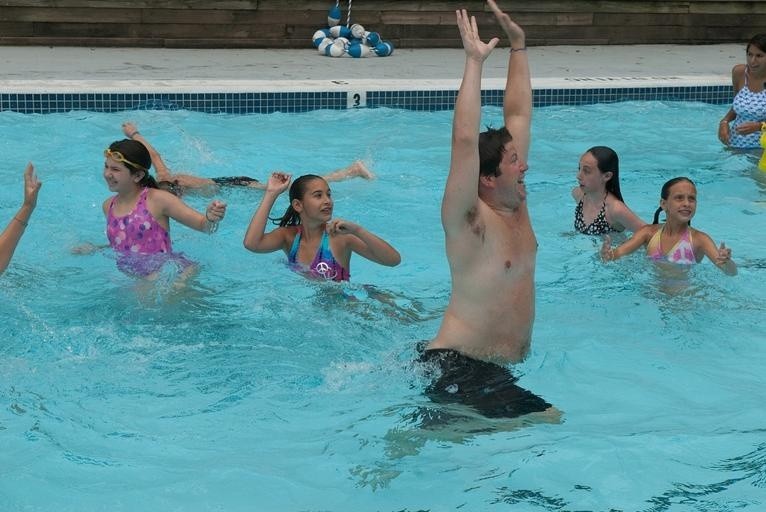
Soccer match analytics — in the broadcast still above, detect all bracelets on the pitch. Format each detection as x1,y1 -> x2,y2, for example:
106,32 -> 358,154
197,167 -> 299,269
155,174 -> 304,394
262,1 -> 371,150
510,48 -> 527,55
15,217 -> 28,227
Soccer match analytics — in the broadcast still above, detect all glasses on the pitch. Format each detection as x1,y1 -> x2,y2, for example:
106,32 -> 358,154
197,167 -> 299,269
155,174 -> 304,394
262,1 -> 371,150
103,149 -> 151,173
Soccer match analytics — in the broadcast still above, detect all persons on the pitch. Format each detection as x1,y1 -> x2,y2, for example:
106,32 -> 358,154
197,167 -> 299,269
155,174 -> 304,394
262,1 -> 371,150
571,146 -> 647,235
718,32 -> 765,148
244,171 -> 401,283
0,164 -> 42,276
419,1 -> 564,427
602,177 -> 737,277
73,140 -> 227,299
123,122 -> 376,197
